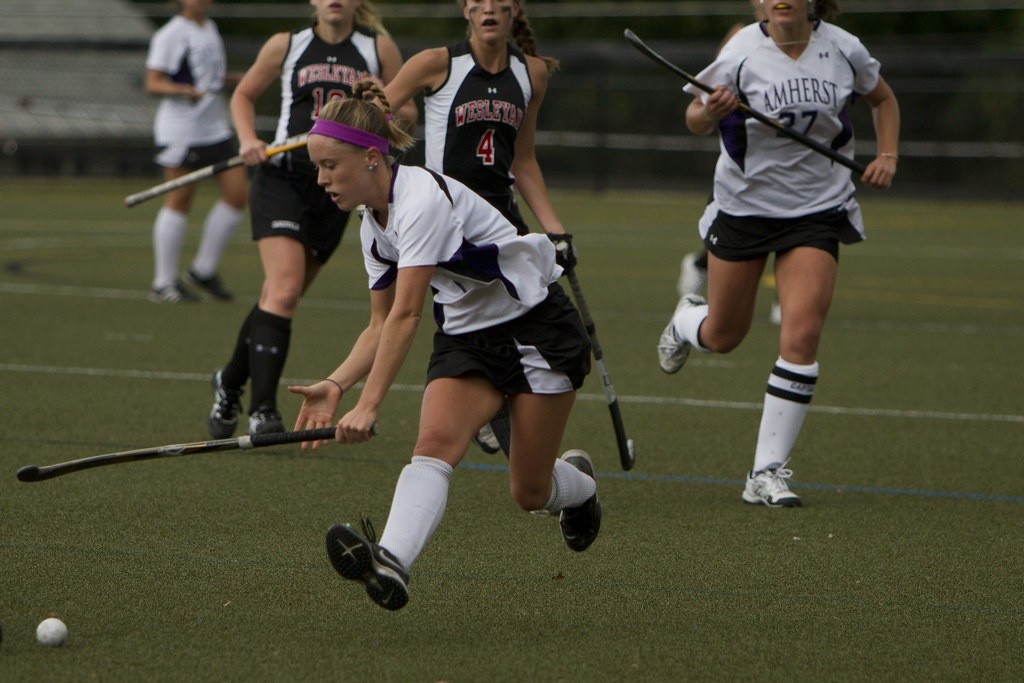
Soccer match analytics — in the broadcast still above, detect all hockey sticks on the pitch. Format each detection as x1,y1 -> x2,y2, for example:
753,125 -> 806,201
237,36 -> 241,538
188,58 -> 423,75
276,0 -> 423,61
554,239 -> 636,471
16,421 -> 381,482
124,133 -> 311,209
623,27 -> 866,175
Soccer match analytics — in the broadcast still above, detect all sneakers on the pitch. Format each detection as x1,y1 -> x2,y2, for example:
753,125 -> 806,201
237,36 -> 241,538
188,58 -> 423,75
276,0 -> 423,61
770,301 -> 782,325
742,457 -> 802,510
559,449 -> 601,552
326,517 -> 410,611
472,423 -> 501,455
181,265 -> 233,303
677,253 -> 706,298
247,402 -> 284,434
147,279 -> 200,306
658,294 -> 705,374
208,368 -> 245,440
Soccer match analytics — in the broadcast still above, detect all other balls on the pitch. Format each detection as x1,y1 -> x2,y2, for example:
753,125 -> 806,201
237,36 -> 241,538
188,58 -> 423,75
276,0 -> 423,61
36,618 -> 69,647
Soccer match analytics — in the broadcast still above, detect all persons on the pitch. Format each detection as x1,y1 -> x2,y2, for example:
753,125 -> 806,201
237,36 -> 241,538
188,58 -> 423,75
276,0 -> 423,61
206,0 -> 579,454
145,0 -> 249,305
288,80 -> 601,611
657,0 -> 900,508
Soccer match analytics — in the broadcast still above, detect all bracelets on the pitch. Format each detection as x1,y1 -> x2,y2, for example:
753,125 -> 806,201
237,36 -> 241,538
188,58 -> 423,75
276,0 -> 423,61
877,153 -> 898,158
702,109 -> 720,122
324,379 -> 343,397
